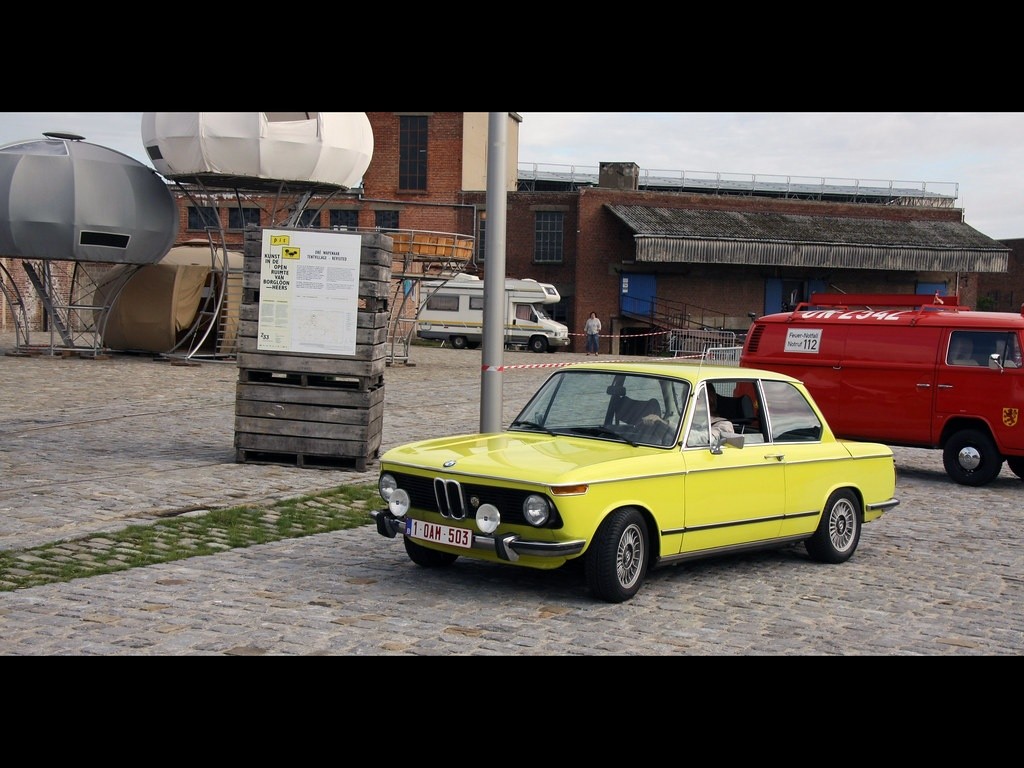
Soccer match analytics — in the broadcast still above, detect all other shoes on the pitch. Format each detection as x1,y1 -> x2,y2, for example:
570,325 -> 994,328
595,352 -> 598,356
587,353 -> 590,355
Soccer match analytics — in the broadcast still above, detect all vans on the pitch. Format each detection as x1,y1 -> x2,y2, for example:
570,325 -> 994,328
732,302 -> 1024,487
413,273 -> 571,354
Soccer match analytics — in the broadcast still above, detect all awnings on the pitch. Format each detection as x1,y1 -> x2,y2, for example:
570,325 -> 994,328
603,203 -> 1013,272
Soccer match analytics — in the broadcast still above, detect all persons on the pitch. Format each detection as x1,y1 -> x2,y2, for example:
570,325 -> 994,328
642,383 -> 735,448
583,312 -> 601,356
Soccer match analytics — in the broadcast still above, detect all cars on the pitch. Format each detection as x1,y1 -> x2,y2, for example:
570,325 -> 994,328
368,362 -> 901,603
